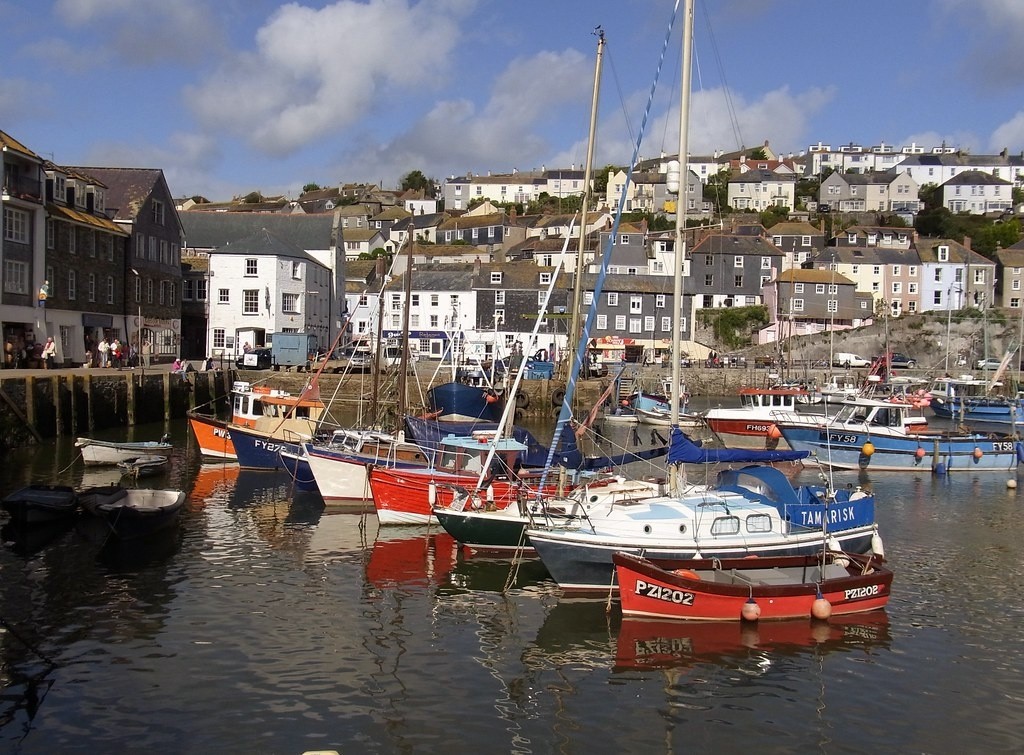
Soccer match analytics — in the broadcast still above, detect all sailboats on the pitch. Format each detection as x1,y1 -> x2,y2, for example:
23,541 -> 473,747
522,0 -> 878,596
226,285 -> 388,471
782,295 -> 1024,423
272,205 -> 417,493
432,23 -> 663,553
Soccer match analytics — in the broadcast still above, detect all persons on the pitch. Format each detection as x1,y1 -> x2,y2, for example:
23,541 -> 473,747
642,350 -> 650,367
110,339 -> 119,368
142,341 -> 152,369
713,352 -> 719,368
129,342 -> 138,370
44,337 -> 57,370
206,357 -> 218,377
620,352 -> 627,367
243,342 -> 252,354
98,338 -> 109,368
200,357 -> 207,370
181,358 -> 198,372
173,359 -> 188,382
115,341 -> 123,371
708,350 -> 714,368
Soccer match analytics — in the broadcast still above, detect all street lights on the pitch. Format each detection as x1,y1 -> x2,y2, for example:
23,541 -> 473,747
131,267 -> 142,375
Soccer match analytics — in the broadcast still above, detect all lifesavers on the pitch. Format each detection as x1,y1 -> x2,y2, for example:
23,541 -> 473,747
674,570 -> 701,580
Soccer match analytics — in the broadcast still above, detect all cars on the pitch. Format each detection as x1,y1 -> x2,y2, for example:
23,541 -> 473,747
872,353 -> 917,369
972,357 -> 1012,372
310,351 -> 351,374
235,348 -> 271,370
832,352 -> 872,368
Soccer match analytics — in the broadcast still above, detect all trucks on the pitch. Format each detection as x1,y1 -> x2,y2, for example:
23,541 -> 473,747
270,331 -> 317,373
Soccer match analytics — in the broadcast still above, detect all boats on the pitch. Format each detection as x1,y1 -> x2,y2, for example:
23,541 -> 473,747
775,395 -> 1019,473
610,545 -> 894,622
78,482 -> 128,517
186,382 -> 325,460
75,437 -> 174,466
612,607 -> 896,676
361,427 -> 622,525
99,488 -> 186,519
1,483 -> 76,524
699,387 -> 833,451
598,365 -> 723,428
303,426 -> 544,505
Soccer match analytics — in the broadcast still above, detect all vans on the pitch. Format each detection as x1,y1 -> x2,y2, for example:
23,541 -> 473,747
373,344 -> 414,374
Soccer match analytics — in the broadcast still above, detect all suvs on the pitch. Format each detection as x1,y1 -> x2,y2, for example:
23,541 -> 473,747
335,348 -> 372,373
